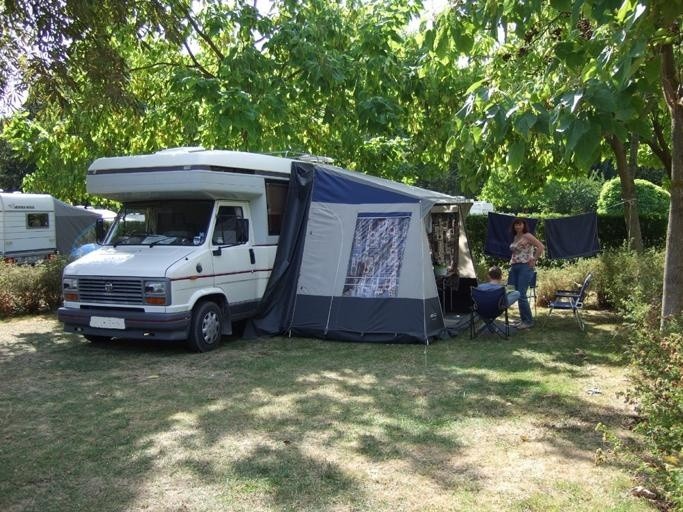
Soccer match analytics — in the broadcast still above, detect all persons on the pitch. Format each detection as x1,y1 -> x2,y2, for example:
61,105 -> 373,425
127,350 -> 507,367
477,266 -> 520,333
509,218 -> 545,329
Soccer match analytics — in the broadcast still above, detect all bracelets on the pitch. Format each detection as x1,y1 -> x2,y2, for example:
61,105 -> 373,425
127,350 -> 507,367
533,257 -> 539,261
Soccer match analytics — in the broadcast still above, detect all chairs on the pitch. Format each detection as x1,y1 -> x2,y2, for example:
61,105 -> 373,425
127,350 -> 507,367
469,286 -> 509,340
536,273 -> 593,334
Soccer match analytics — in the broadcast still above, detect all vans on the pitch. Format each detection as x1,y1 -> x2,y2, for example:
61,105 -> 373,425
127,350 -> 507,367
59,145 -> 317,348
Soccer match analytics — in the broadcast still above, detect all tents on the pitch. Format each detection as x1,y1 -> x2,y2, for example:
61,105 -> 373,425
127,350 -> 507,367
249,161 -> 477,343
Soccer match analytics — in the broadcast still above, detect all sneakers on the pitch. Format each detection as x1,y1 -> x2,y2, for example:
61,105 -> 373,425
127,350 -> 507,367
513,321 -> 534,329
